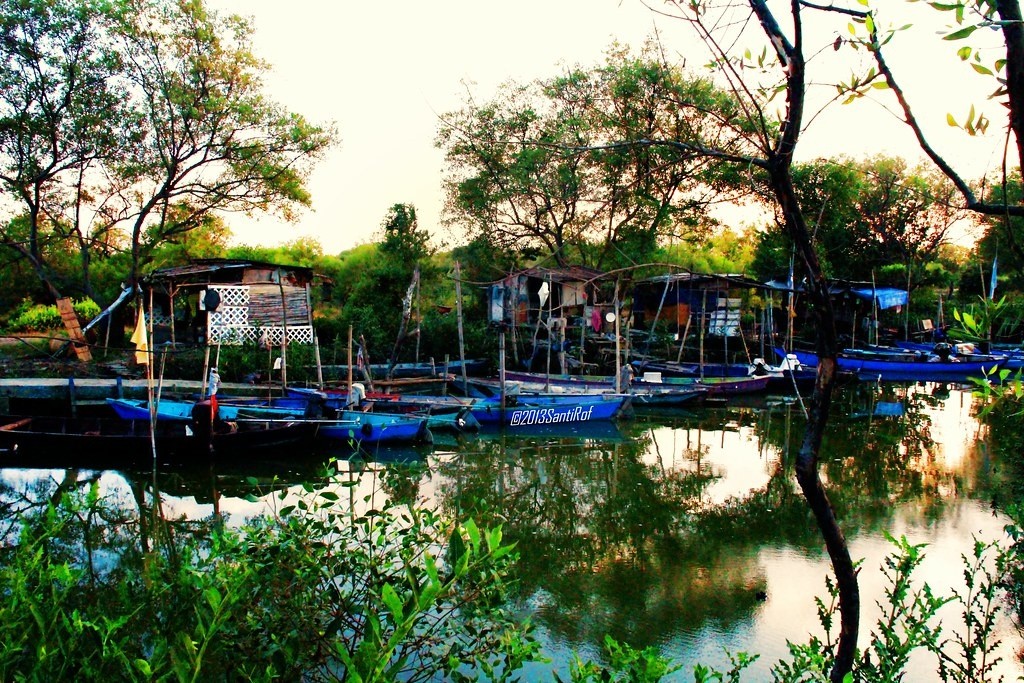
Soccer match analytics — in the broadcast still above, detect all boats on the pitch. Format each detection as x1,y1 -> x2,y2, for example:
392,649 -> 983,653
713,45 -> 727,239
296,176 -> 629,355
276,253 -> 1023,434
0,412 -> 153,467
105,258 -> 428,444
